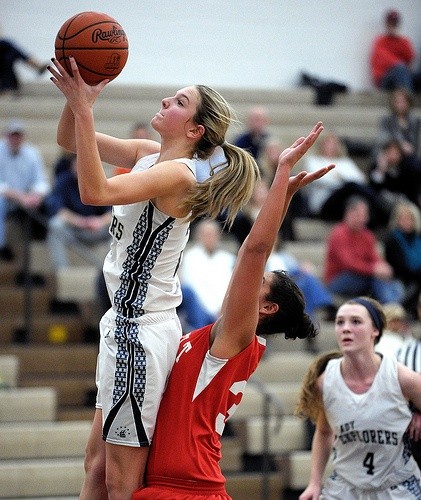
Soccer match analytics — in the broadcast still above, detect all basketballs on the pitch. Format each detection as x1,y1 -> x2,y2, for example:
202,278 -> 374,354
54,12 -> 129,84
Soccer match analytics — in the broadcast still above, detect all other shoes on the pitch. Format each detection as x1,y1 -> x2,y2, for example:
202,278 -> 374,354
384,303 -> 405,320
0,244 -> 14,260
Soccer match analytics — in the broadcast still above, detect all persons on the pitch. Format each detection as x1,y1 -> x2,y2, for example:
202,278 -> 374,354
129,121 -> 335,500
47,55 -> 260,500
296,296 -> 421,500
0,89 -> 420,329
371,8 -> 420,92
375,297 -> 421,468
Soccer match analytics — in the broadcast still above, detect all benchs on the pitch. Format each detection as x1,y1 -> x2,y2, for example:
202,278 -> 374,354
1,83 -> 421,500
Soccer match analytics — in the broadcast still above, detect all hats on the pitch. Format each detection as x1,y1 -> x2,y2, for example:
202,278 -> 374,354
7,121 -> 24,134
